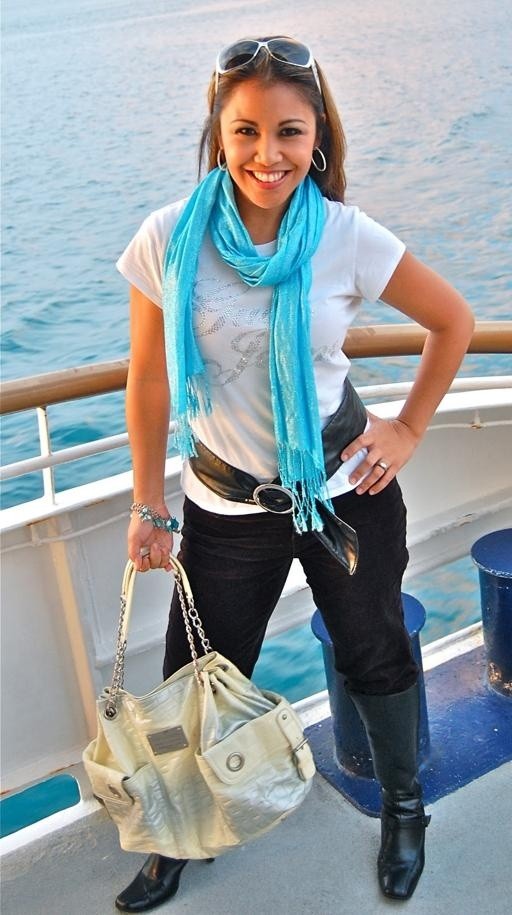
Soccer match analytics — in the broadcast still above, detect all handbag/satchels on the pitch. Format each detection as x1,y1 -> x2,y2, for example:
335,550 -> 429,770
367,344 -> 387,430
81,653 -> 315,861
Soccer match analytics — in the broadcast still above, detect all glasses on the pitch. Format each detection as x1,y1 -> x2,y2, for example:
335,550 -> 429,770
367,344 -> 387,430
215,37 -> 323,97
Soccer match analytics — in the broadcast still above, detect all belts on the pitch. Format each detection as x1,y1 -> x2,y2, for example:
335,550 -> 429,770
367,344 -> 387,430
188,375 -> 367,577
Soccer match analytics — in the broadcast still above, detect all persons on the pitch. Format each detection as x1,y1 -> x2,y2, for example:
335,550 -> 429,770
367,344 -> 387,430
114,34 -> 476,913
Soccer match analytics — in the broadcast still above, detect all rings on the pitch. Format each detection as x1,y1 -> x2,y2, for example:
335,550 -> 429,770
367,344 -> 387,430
376,462 -> 387,472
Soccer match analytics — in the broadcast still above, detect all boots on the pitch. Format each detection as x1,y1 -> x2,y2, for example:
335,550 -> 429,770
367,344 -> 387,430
114,852 -> 215,913
345,682 -> 430,900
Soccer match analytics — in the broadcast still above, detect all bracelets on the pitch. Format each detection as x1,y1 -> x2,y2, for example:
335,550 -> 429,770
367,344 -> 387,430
130,502 -> 180,535
